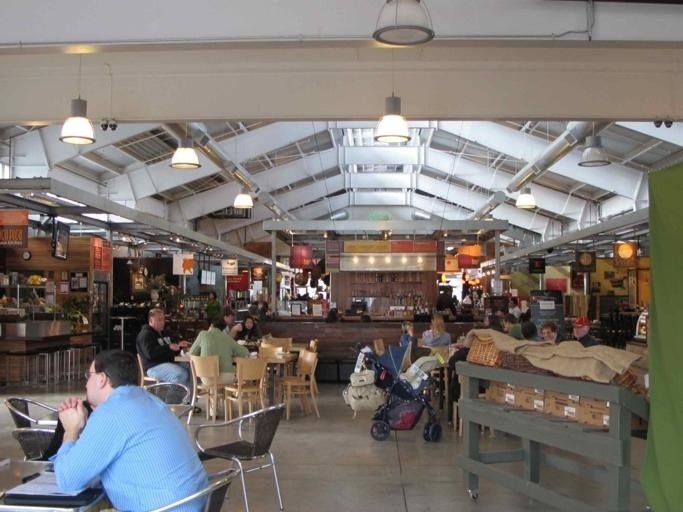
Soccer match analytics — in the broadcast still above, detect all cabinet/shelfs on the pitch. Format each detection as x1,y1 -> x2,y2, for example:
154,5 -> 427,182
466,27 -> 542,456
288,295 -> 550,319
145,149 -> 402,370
448,356 -> 650,512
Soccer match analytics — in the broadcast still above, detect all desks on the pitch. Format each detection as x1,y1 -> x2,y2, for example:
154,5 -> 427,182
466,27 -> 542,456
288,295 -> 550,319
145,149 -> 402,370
0,458 -> 106,511
37,405 -> 192,427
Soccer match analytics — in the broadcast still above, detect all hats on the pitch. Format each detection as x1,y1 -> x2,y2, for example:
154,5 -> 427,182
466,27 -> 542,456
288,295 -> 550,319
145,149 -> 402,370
572,318 -> 592,327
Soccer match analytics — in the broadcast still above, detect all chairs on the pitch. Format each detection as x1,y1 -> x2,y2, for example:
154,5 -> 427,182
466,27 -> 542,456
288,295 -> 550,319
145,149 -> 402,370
5,396 -> 60,428
372,335 -> 498,444
136,332 -> 323,423
195,404 -> 287,511
11,429 -> 56,462
155,468 -> 242,512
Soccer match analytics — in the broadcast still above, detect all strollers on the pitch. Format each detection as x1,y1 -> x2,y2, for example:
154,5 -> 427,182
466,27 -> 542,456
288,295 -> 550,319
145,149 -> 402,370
363,336 -> 441,443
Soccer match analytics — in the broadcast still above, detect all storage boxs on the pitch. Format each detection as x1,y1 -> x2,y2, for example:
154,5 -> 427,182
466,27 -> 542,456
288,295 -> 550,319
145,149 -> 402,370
481,379 -> 651,432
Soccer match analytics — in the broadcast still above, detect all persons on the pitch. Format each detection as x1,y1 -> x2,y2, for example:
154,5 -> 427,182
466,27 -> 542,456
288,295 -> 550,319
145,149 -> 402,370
221,311 -> 243,338
137,308 -> 201,412
48,349 -> 209,512
190,318 -> 250,419
317,294 -> 323,300
249,293 -> 268,319
400,286 -> 597,428
205,291 -> 221,323
211,306 -> 230,323
290,293 -> 311,301
240,315 -> 257,338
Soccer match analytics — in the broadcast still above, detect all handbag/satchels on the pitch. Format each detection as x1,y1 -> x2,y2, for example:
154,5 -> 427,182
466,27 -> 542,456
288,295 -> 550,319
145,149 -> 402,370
343,370 -> 386,411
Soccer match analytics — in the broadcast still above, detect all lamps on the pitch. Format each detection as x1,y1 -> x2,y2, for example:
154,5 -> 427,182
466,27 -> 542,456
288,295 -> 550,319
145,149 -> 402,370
55,43 -> 255,210
371,1 -> 435,46
371,47 -> 411,144
485,115 -> 640,273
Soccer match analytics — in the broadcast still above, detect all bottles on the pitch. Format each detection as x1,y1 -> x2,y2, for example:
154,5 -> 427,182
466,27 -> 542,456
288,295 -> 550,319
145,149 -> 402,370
387,288 -> 428,319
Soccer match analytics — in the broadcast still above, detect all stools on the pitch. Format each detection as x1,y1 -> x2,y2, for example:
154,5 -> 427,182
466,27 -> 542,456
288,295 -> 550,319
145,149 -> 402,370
0,341 -> 103,387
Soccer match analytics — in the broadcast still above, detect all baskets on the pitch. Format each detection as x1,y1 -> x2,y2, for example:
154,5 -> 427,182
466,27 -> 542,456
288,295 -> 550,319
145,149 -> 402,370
466,335 -> 551,373
611,369 -> 648,395
